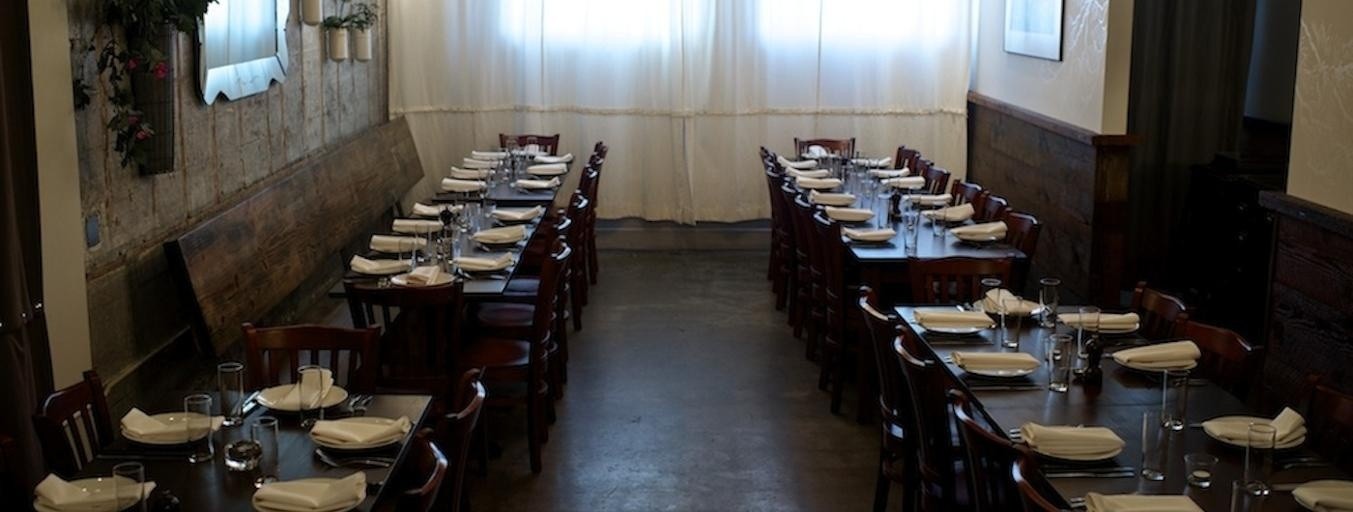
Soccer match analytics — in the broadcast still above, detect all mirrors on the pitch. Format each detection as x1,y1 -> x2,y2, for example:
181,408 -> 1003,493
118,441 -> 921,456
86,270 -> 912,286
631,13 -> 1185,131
194,0 -> 290,107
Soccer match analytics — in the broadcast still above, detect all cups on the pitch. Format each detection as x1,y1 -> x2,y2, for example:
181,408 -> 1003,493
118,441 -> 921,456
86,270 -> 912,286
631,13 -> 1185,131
775,144 -> 1007,253
981,279 -> 1001,326
1141,409 -> 1173,480
1243,422 -> 1276,496
349,196 -> 542,288
1077,306 -> 1100,359
185,394 -> 214,463
298,365 -> 324,429
431,135 -> 575,202
1048,332 -> 1072,393
113,461 -> 146,511
217,361 -> 244,427
1185,454 -> 1219,487
999,294 -> 1023,347
1161,368 -> 1190,431
251,417 -> 282,488
1039,278 -> 1061,329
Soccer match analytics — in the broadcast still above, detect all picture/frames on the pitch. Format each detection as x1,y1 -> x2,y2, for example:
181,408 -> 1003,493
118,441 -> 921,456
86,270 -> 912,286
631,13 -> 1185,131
1002,0 -> 1066,62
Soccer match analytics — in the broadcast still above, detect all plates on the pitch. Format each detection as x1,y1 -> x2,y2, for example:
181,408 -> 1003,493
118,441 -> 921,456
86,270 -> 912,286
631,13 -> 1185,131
311,417 -> 403,449
1033,447 -> 1123,462
256,384 -> 348,411
1115,352 -> 1197,373
973,298 -> 1044,316
252,477 -> 365,511
1073,322 -> 1139,332
965,366 -> 1034,377
121,411 -> 210,445
921,323 -> 984,334
33,478 -> 140,511
1204,416 -> 1306,449
1294,480 -> 1353,512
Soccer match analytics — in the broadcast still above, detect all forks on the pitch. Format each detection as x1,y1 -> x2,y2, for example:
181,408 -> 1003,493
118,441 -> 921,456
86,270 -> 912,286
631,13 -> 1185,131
355,395 -> 373,411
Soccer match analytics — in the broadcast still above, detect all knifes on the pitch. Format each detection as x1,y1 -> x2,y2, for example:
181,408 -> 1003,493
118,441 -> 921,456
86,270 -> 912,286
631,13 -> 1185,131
243,393 -> 260,413
969,385 -> 1045,391
1046,472 -> 1135,478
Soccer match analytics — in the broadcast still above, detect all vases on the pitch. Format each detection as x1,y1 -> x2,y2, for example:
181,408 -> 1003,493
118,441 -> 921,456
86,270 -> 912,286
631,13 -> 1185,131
129,20 -> 174,177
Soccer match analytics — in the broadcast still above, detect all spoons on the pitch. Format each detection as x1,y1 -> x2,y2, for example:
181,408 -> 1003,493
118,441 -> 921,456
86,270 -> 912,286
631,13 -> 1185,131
315,447 -> 390,468
254,475 -> 280,488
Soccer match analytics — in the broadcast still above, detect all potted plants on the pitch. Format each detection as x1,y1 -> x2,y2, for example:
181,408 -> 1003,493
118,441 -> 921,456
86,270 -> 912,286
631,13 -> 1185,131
322,0 -> 379,63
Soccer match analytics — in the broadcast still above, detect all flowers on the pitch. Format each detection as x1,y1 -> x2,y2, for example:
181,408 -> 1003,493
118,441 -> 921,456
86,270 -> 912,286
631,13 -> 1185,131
70,0 -> 220,169
